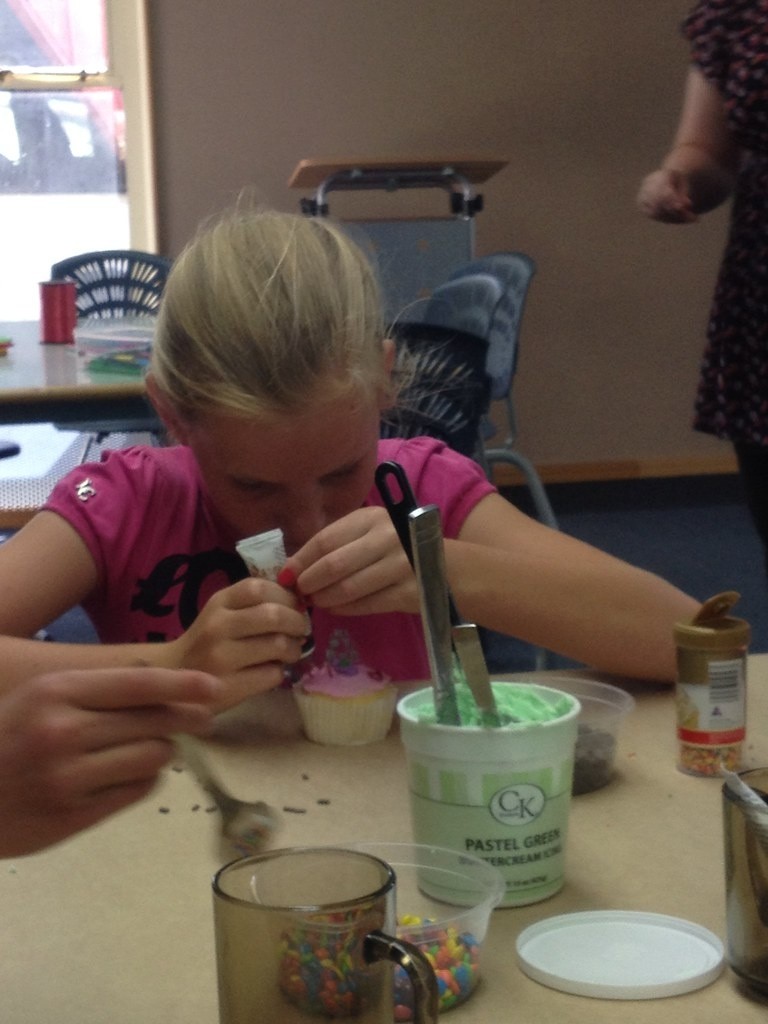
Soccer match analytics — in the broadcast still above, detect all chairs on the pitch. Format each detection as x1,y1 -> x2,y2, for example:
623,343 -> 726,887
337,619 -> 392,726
421,272 -> 559,531
444,249 -> 538,447
50,248 -> 170,464
379,320 -> 493,458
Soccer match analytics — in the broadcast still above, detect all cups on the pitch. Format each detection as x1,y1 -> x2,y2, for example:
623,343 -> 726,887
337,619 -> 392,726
210,845 -> 438,1024
396,682 -> 582,909
722,767 -> 768,1006
40,280 -> 76,343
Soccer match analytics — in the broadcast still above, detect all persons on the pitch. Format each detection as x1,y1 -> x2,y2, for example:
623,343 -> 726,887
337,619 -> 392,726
0,208 -> 705,863
639,0 -> 768,597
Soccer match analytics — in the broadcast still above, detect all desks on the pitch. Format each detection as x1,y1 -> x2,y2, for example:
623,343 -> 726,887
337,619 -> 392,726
0,315 -> 165,539
0,651 -> 768,1024
288,152 -> 510,264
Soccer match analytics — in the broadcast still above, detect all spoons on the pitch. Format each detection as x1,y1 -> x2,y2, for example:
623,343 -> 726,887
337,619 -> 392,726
134,658 -> 278,849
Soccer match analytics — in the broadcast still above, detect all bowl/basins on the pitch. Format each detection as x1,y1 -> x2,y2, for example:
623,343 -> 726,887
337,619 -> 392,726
490,676 -> 636,796
250,842 -> 505,1024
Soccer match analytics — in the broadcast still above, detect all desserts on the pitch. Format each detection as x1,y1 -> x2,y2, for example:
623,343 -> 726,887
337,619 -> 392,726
293,654 -> 397,746
